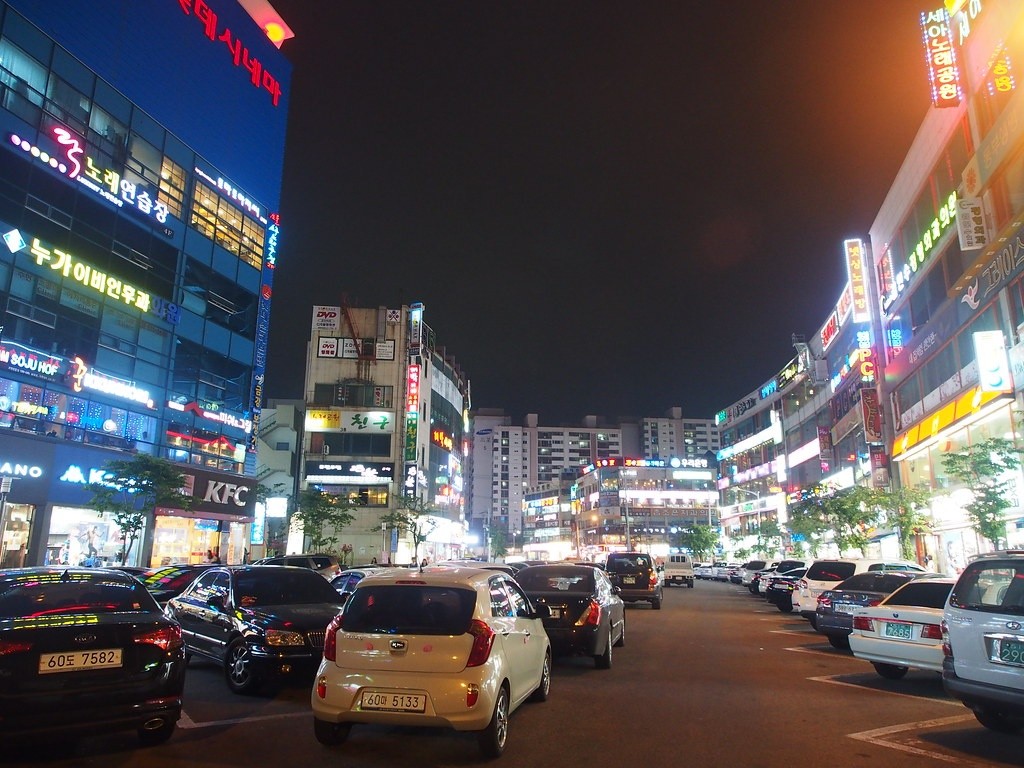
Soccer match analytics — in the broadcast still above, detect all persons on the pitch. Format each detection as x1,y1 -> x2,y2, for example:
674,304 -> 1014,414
207,546 -> 220,564
78,526 -> 104,559
924,555 -> 935,571
661,562 -> 664,571
371,557 -> 377,563
408,557 -> 428,568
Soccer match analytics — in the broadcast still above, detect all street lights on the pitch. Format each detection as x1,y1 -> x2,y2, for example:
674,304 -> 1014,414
729,487 -> 761,560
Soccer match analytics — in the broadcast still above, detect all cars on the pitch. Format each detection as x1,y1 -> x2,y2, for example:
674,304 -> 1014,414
814,570 -> 949,650
433,556 -> 605,578
326,567 -> 396,600
0,566 -> 188,749
161,564 -> 346,697
99,564 -> 155,577
312,566 -> 554,760
511,564 -> 626,669
133,564 -> 228,609
691,557 -> 844,612
846,576 -> 1011,679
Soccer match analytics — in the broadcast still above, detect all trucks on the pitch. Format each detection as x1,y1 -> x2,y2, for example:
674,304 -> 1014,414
661,553 -> 695,588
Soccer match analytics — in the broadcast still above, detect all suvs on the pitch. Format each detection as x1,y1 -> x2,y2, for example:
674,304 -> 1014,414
790,555 -> 928,630
254,553 -> 341,582
938,548 -> 1024,736
604,552 -> 664,610
